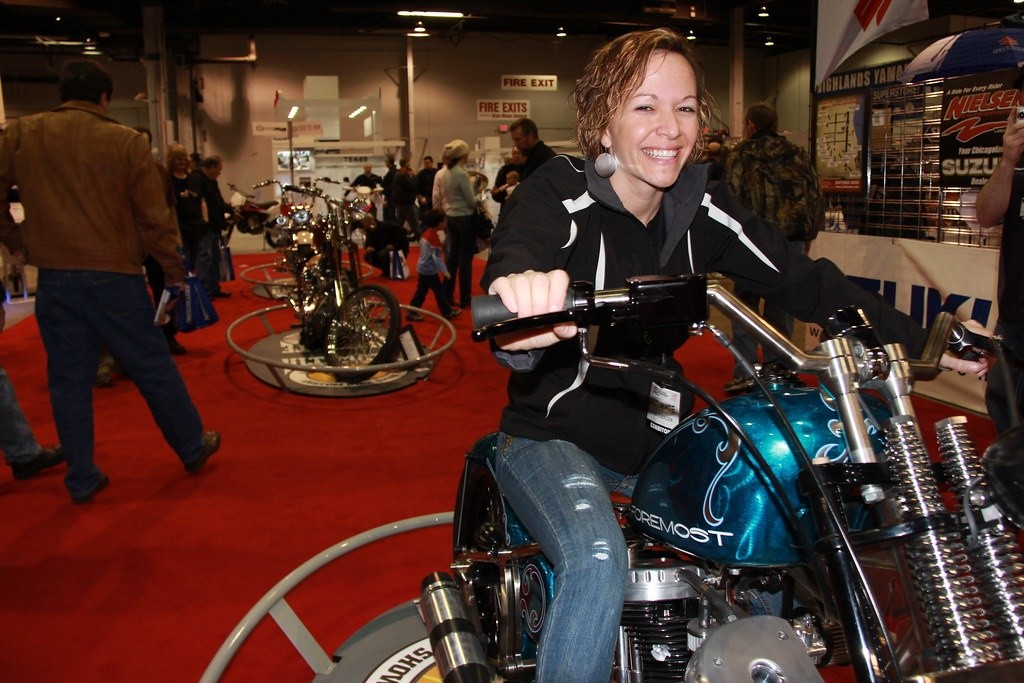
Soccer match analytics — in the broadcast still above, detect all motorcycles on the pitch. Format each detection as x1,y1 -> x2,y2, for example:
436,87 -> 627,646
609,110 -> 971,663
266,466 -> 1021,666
419,261 -> 1022,683
254,173 -> 407,386
215,176 -> 284,247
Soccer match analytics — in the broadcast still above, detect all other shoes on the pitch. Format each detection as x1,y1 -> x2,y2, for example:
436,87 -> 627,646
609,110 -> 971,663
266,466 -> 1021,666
722,377 -> 755,391
452,302 -> 471,309
406,314 -> 424,321
184,431 -> 221,473
380,273 -> 390,276
13,444 -> 65,480
442,309 -> 462,319
73,473 -> 109,503
169,342 -> 185,354
218,291 -> 231,298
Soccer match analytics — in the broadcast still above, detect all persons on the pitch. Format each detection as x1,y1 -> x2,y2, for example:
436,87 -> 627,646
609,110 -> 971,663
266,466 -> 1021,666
0,59 -> 221,505
87,126 -> 239,389
0,280 -> 64,480
477,27 -> 1004,683
343,120 -> 559,320
974,106 -> 1024,462
695,102 -> 822,394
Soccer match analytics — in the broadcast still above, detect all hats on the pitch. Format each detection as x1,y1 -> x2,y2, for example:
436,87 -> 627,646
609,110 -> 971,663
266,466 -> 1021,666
442,139 -> 471,163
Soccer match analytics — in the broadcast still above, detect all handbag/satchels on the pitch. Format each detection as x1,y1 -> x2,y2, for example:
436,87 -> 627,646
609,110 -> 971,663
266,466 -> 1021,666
176,274 -> 218,332
474,198 -> 498,253
218,237 -> 235,282
390,251 -> 410,281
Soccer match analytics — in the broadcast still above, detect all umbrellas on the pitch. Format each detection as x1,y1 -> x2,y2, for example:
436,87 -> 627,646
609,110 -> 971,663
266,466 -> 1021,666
897,27 -> 1024,83
